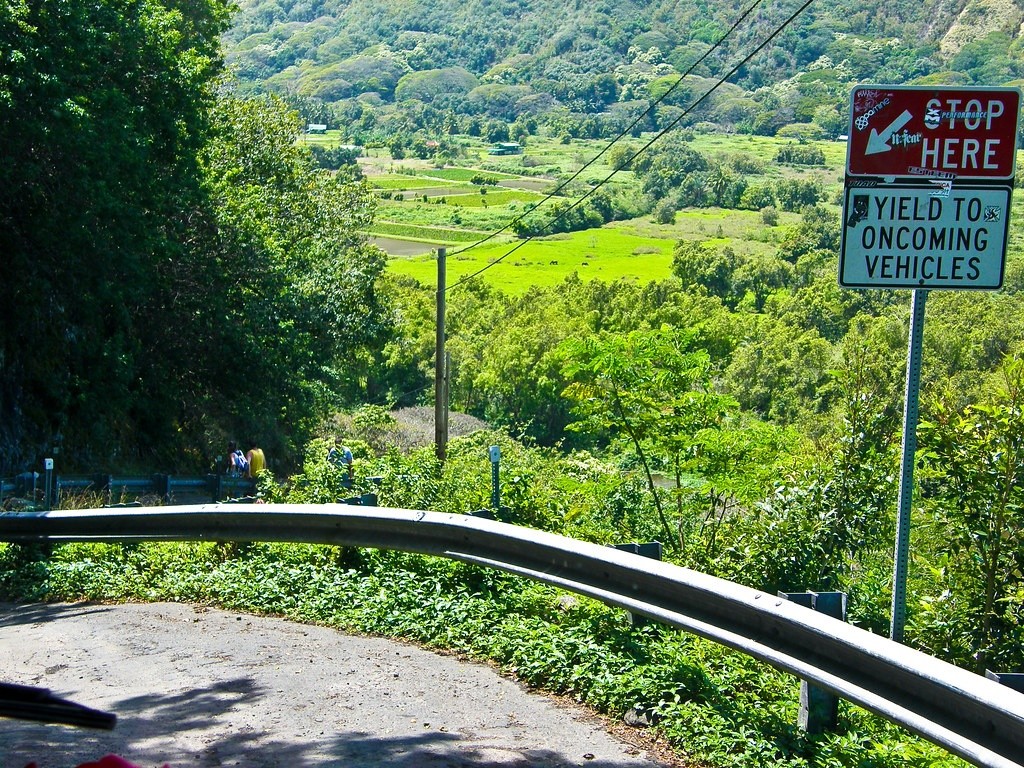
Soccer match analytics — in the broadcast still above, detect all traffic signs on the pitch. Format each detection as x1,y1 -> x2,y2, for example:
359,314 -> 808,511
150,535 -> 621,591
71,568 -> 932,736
838,81 -> 1020,180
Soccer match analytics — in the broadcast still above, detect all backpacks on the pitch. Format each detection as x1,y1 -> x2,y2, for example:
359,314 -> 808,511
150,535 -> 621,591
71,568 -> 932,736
232,450 -> 249,472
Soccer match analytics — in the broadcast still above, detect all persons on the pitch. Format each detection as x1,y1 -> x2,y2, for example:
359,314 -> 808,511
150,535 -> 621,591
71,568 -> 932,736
329,439 -> 352,488
229,441 -> 266,478
